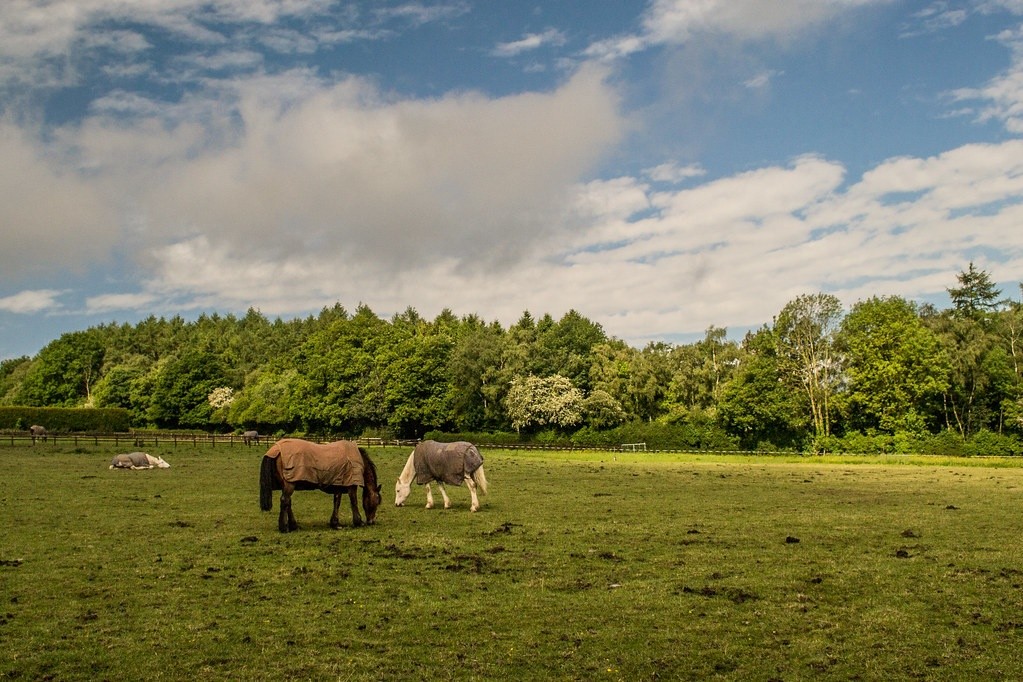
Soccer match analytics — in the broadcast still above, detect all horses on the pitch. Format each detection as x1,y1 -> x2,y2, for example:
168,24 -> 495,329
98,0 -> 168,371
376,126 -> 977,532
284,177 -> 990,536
394,440 -> 488,512
241,430 -> 259,445
109,452 -> 172,471
260,439 -> 383,532
31,424 -> 48,442
